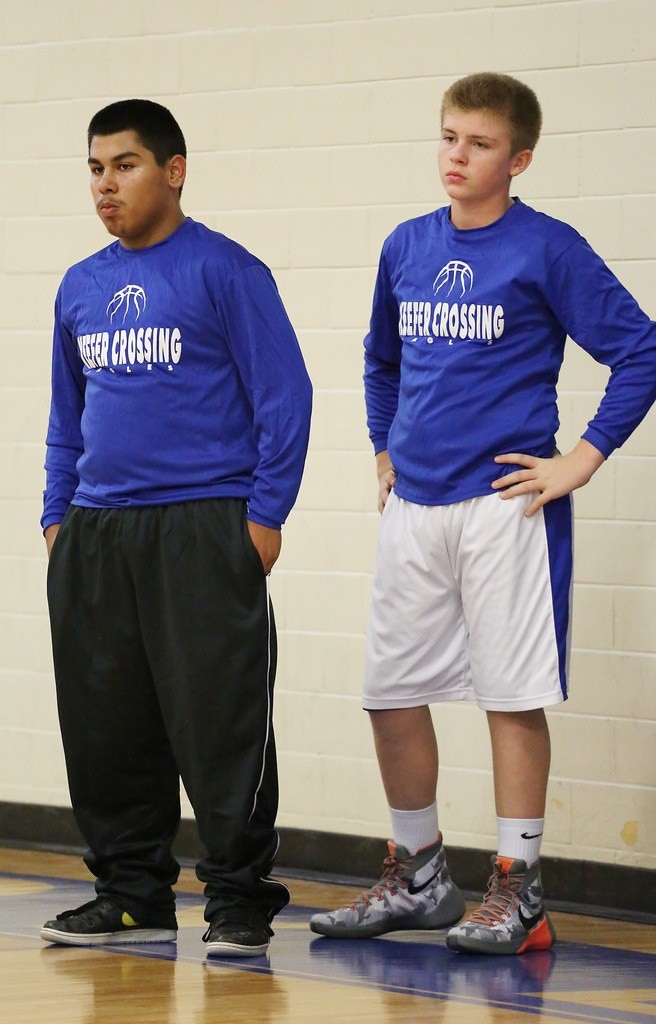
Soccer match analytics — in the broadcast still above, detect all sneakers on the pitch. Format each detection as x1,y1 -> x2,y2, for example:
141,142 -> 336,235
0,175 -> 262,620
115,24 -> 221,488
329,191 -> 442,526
203,912 -> 276,957
307,828 -> 469,939
445,853 -> 558,957
38,896 -> 178,946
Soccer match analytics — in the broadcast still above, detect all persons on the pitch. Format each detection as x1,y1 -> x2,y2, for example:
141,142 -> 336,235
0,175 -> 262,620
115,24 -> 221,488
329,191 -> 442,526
39,96 -> 312,957
309,75 -> 656,954
